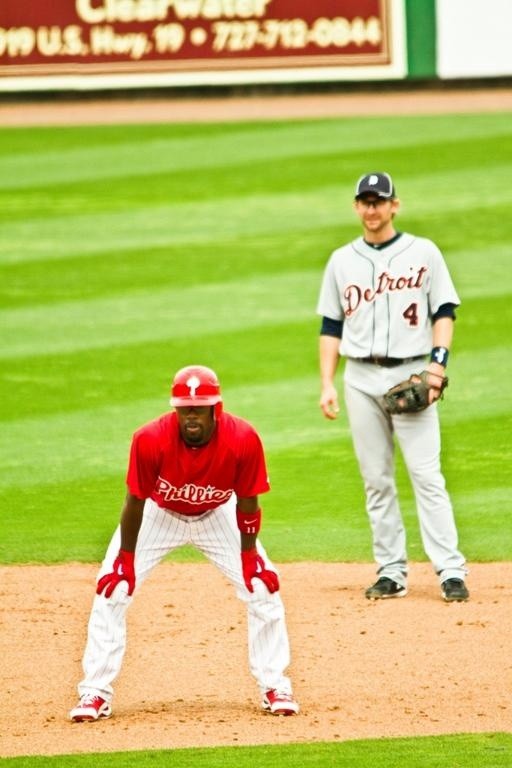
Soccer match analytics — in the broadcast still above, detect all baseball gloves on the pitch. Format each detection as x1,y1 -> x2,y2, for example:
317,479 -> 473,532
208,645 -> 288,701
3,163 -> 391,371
383,370 -> 449,414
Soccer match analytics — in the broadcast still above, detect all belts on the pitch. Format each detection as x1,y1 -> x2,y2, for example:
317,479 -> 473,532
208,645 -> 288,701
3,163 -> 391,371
358,356 -> 423,367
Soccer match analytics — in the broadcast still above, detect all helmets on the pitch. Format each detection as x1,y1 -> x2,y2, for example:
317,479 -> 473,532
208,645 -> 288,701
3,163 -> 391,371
170,365 -> 223,423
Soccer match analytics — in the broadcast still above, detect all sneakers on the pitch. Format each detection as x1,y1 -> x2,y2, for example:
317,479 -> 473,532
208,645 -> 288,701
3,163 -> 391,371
440,577 -> 468,602
365,577 -> 407,599
70,694 -> 112,722
262,688 -> 299,716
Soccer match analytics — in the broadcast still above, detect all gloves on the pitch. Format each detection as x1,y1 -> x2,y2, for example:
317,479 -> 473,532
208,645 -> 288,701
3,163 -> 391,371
96,548 -> 135,599
241,548 -> 279,594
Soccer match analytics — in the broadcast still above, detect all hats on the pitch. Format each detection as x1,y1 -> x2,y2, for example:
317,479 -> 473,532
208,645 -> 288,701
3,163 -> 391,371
354,171 -> 397,202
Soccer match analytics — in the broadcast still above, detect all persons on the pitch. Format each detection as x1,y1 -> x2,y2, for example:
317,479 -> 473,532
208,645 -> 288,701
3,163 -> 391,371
318,169 -> 469,602
69,364 -> 300,722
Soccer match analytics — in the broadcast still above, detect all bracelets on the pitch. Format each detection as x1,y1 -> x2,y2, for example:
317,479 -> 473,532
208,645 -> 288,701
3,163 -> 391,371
430,347 -> 449,368
